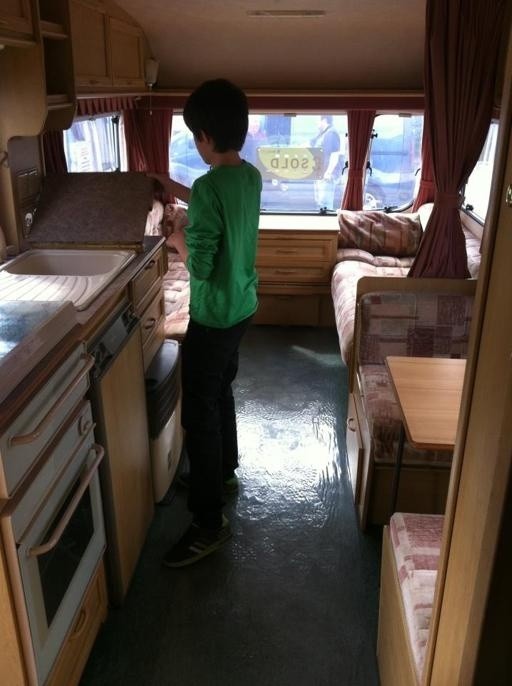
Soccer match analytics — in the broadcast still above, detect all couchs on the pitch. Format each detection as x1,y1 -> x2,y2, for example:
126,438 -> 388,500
331,200 -> 485,373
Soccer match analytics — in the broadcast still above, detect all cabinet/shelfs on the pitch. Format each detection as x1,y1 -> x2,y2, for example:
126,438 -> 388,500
73,2 -> 149,91
130,248 -> 168,368
251,214 -> 339,330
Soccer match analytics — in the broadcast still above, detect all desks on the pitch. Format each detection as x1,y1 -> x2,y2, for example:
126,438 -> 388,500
384,356 -> 468,449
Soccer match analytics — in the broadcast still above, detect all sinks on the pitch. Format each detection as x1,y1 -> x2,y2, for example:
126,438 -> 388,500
0,248 -> 138,277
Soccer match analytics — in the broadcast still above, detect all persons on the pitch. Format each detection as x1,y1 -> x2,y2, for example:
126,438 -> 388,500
169,118 -> 269,177
300,115 -> 340,211
143,77 -> 262,569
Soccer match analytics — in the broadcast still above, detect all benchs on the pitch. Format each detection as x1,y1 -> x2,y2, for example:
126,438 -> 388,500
346,278 -> 477,519
376,513 -> 444,686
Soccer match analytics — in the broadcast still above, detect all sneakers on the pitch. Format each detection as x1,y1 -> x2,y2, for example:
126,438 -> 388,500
177,472 -> 239,495
162,514 -> 233,567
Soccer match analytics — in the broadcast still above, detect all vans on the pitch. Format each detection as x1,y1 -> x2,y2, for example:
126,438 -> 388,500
171,114 -> 416,210
